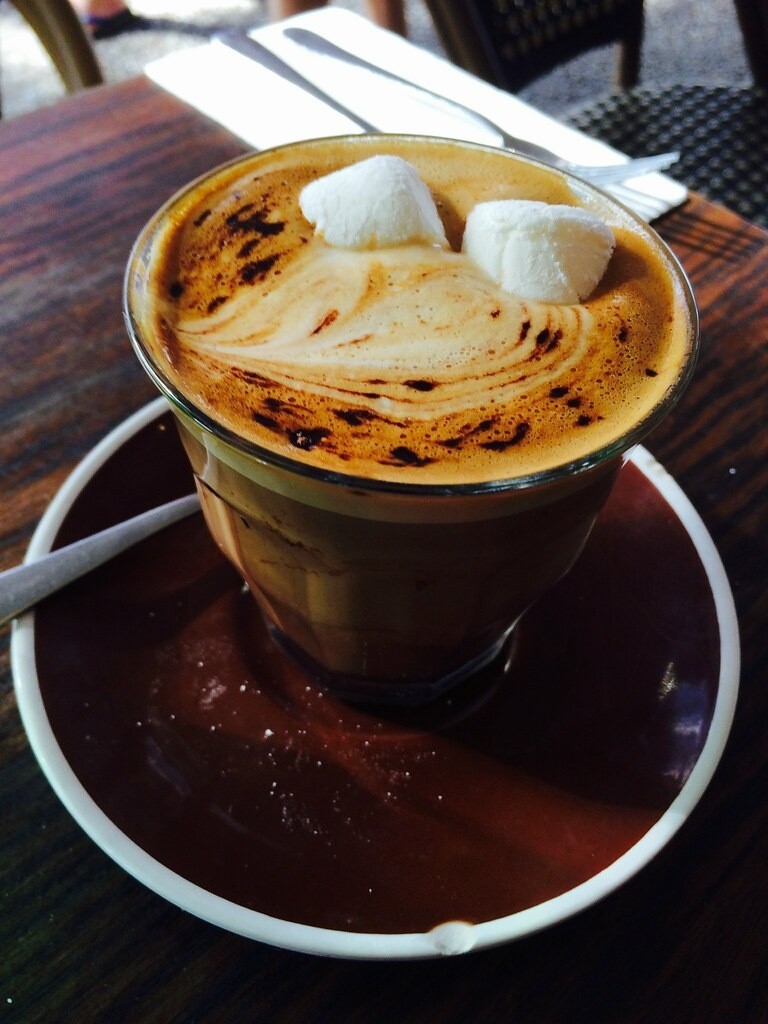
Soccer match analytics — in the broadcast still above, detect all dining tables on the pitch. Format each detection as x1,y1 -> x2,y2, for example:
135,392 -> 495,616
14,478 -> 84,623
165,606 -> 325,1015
1,0 -> 768,1024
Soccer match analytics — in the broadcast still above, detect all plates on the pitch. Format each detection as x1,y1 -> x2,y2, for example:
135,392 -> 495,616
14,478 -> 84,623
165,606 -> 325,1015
12,392 -> 742,959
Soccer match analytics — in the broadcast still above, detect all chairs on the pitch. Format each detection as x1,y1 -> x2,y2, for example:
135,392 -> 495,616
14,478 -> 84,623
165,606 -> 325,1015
425,0 -> 768,239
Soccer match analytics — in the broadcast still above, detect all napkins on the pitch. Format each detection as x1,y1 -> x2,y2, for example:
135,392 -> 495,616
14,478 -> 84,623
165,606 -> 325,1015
145,6 -> 691,225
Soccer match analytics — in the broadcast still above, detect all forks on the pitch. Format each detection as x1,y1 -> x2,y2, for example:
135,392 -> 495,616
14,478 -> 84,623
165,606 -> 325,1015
284,25 -> 680,192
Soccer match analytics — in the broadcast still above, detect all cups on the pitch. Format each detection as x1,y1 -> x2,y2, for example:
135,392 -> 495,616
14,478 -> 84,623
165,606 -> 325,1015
121,132 -> 703,691
